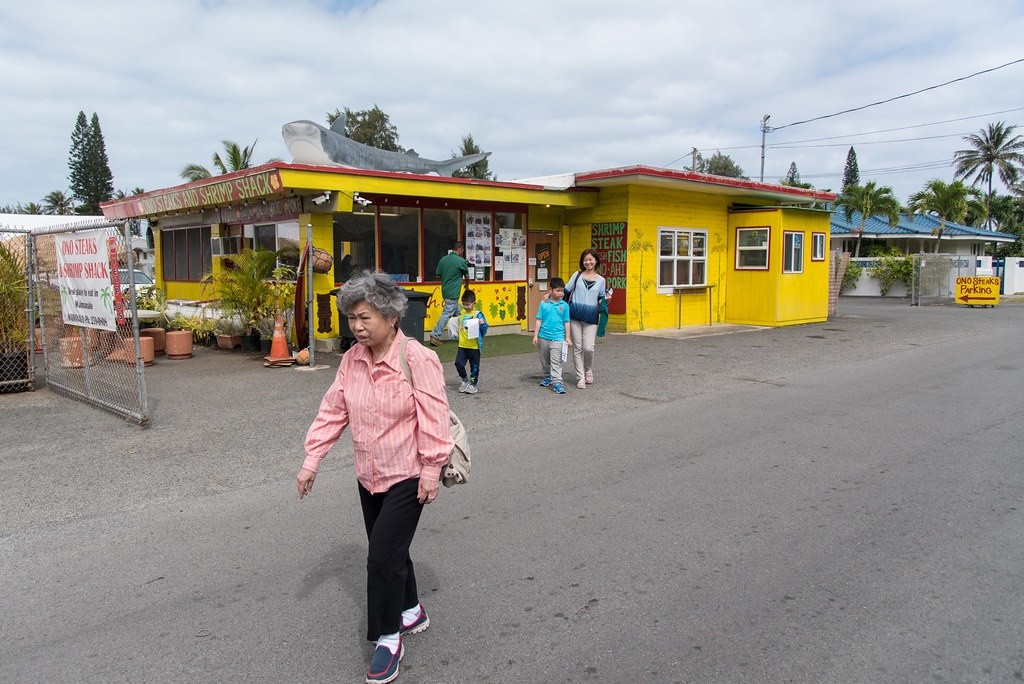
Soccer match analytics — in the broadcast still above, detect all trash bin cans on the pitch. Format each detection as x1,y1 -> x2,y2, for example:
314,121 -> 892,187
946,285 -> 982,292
329,288 -> 358,352
401,290 -> 433,345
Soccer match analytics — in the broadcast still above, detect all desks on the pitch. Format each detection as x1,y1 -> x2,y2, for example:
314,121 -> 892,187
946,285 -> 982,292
114,309 -> 161,318
673,285 -> 716,329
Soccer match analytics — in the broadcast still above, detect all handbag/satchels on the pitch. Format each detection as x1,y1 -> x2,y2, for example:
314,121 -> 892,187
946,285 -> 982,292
447,316 -> 459,341
562,271 -> 582,304
399,336 -> 471,488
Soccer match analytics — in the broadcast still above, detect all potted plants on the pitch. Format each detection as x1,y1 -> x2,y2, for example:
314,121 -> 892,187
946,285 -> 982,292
167,247 -> 296,353
0,241 -> 29,394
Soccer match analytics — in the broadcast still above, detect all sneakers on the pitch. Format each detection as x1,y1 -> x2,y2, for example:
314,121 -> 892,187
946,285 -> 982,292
429,336 -> 442,345
577,379 -> 585,389
540,377 -> 553,387
365,635 -> 404,684
552,382 -> 566,393
585,369 -> 593,384
458,377 -> 470,392
465,384 -> 477,394
373,604 -> 430,645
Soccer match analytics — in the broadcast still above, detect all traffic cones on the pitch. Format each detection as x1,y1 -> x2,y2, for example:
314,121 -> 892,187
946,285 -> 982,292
263,308 -> 297,367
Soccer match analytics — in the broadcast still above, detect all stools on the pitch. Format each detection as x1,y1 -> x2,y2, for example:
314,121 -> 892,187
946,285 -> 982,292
35,324 -> 192,367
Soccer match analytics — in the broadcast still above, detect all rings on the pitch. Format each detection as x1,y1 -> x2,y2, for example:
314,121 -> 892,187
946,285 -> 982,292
428,496 -> 435,500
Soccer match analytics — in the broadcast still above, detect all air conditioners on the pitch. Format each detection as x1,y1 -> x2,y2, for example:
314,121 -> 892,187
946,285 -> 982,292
210,236 -> 240,257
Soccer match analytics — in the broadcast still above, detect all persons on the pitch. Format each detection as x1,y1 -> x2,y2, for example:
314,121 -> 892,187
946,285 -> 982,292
340,254 -> 353,282
296,269 -> 471,684
544,249 -> 613,389
449,290 -> 489,394
429,241 -> 469,346
531,277 -> 572,394
